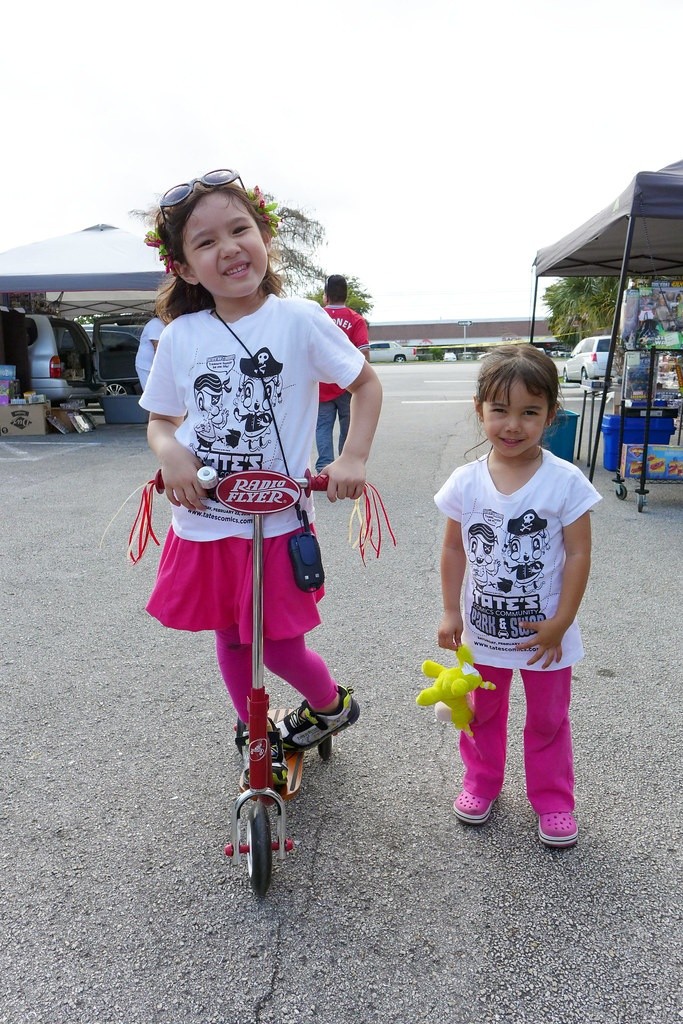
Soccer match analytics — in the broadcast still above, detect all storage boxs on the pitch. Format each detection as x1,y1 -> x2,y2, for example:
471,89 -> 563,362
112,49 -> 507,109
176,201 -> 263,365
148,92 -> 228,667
0,401 -> 52,438
601,413 -> 676,472
539,408 -> 578,466
101,395 -> 149,424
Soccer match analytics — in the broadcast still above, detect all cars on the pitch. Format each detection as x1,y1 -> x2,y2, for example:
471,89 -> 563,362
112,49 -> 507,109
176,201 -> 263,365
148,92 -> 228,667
369,340 -> 418,364
443,347 -> 570,361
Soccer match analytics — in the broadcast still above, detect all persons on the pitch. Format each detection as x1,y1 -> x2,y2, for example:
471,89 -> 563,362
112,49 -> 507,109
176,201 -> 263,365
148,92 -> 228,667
136,170 -> 383,786
431,343 -> 605,846
136,315 -> 166,394
316,274 -> 371,475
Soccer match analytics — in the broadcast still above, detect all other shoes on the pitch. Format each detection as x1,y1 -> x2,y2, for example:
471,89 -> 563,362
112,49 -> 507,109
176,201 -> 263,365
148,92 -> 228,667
452,789 -> 500,825
538,812 -> 579,847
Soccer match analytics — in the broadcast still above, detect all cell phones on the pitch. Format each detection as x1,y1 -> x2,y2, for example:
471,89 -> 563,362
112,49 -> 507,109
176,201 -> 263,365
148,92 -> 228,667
288,509 -> 325,592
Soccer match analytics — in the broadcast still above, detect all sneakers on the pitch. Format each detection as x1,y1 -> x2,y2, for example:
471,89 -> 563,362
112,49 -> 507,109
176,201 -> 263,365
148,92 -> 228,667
235,716 -> 289,786
274,683 -> 361,752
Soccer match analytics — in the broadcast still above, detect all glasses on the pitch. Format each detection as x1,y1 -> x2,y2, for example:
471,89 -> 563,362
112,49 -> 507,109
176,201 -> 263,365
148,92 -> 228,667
159,168 -> 248,230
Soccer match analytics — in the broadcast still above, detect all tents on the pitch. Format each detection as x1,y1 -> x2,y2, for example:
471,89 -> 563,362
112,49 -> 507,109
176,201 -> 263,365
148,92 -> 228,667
527,160 -> 683,483
0,223 -> 176,318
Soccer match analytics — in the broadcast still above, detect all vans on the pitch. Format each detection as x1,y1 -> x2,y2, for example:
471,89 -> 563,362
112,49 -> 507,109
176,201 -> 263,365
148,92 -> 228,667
22,314 -> 108,402
562,335 -> 616,383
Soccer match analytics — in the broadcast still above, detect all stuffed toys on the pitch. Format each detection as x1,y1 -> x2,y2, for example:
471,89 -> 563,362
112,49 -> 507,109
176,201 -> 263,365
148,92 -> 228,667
415,644 -> 497,736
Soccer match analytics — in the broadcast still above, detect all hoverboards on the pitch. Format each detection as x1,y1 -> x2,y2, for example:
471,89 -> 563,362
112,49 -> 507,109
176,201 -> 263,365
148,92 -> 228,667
151,465 -> 335,896
